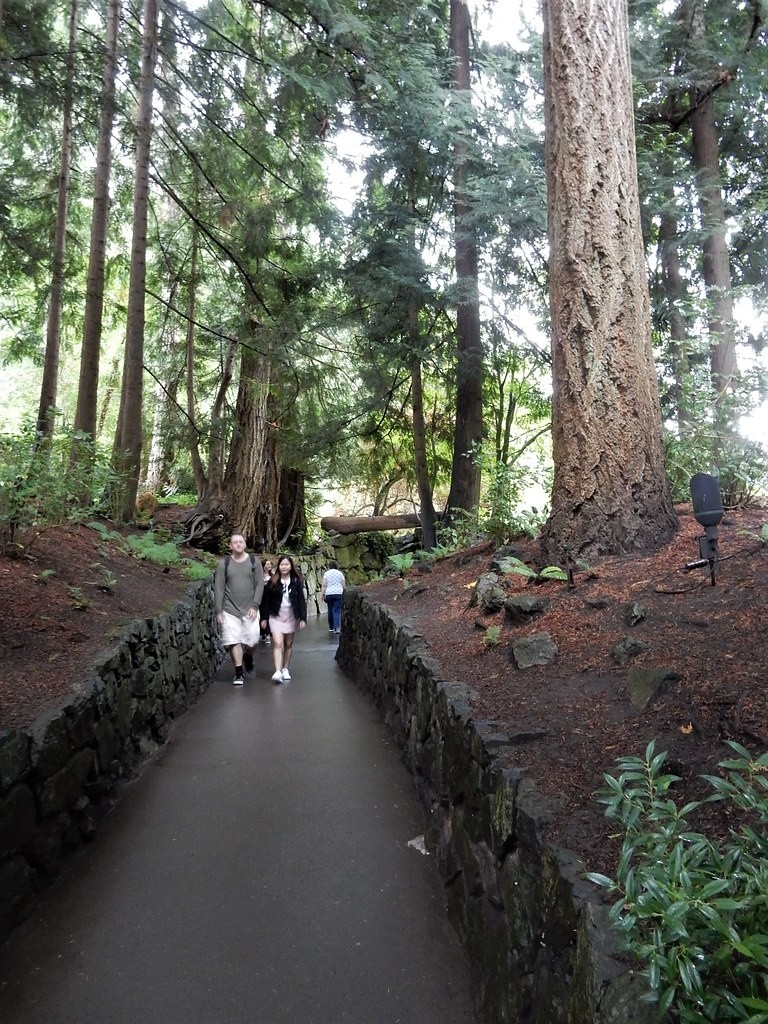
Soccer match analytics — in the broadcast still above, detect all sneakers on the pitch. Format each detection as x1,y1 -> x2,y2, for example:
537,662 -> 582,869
233,673 -> 246,684
271,671 -> 283,684
243,652 -> 256,679
282,668 -> 291,679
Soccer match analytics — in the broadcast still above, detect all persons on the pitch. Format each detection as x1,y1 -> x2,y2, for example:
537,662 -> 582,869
320,560 -> 345,634
214,531 -> 264,685
261,555 -> 307,683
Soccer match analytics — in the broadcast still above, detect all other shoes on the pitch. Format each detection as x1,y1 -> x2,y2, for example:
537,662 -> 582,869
329,629 -> 334,631
262,634 -> 271,644
334,628 -> 340,634
